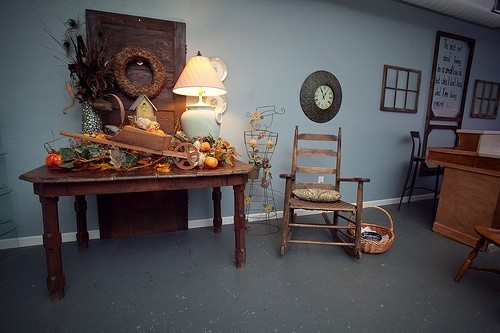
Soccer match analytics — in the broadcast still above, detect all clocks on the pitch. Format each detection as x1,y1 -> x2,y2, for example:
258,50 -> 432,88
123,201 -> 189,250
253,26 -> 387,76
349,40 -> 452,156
300,70 -> 343,123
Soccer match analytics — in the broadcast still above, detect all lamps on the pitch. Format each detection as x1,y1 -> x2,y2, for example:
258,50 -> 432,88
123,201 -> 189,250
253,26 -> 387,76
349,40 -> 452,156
172,55 -> 227,141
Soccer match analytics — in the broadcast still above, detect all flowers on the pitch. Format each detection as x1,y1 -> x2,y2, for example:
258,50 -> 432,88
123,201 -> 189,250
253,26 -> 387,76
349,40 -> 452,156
45,133 -> 242,173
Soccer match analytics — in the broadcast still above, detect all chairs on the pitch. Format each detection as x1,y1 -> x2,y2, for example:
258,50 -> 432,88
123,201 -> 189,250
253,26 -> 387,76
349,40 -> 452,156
455,225 -> 500,282
397,130 -> 440,212
279,125 -> 370,259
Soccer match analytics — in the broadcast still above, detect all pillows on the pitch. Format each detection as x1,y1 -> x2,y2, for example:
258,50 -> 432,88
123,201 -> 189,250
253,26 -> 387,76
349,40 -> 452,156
292,189 -> 342,202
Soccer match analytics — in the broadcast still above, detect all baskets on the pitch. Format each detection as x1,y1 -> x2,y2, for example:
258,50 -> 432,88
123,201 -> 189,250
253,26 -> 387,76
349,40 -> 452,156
346,205 -> 393,254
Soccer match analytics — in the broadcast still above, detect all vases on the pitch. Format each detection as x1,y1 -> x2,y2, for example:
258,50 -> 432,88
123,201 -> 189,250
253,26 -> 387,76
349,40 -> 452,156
82,100 -> 101,135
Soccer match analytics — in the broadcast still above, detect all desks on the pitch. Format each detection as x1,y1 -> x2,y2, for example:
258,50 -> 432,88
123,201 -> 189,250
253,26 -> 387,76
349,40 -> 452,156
18,154 -> 256,303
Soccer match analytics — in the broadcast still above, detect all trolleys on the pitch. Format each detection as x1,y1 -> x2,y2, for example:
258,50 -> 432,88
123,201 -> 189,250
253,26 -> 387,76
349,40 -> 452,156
58,127 -> 201,170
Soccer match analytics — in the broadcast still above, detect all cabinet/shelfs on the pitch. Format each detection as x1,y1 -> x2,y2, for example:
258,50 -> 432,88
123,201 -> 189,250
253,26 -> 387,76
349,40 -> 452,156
426,129 -> 500,251
